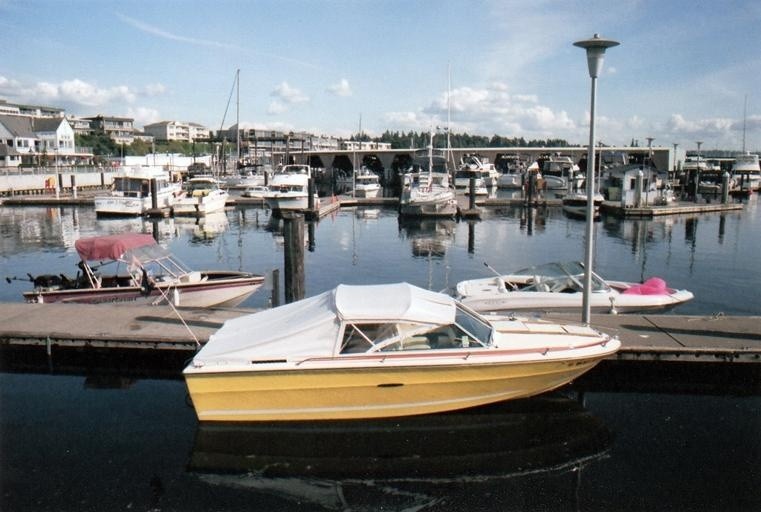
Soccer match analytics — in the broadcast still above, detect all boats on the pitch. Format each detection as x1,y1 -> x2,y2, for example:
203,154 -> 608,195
97,218 -> 181,249
171,172 -> 230,213
177,213 -> 231,246
93,162 -> 185,219
409,218 -> 454,262
190,413 -> 627,511
24,230 -> 268,308
263,215 -> 310,249
455,259 -> 704,313
175,277 -> 626,429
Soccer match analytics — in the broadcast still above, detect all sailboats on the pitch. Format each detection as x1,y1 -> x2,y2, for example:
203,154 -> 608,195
181,68 -> 270,186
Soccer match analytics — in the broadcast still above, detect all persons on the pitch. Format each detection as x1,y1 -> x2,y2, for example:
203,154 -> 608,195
653,174 -> 662,188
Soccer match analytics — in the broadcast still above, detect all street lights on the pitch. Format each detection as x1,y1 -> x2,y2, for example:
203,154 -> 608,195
572,32 -> 620,325
29,145 -> 41,166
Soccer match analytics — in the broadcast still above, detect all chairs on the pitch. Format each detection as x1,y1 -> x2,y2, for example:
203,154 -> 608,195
345,323 -> 431,353
